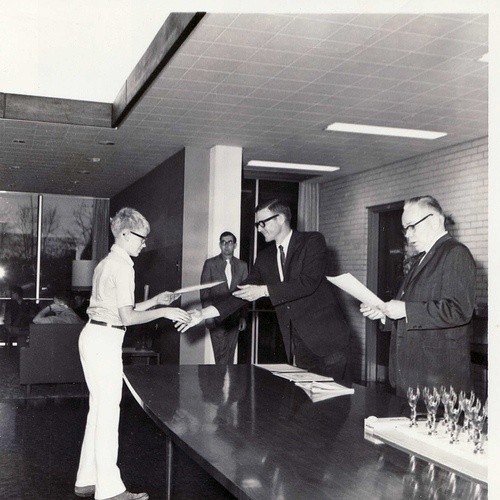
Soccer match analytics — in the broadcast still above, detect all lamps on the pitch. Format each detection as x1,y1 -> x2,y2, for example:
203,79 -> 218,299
71,259 -> 98,292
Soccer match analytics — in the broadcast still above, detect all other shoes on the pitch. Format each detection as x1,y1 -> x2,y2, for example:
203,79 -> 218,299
95,489 -> 149,500
75,484 -> 95,497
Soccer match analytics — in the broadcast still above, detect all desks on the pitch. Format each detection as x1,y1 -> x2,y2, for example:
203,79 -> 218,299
122,364 -> 488,500
122,347 -> 161,366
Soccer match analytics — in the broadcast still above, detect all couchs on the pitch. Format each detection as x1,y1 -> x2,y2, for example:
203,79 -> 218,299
17,323 -> 86,395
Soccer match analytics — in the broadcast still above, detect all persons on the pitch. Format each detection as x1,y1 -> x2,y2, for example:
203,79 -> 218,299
359,195 -> 478,415
4,286 -> 90,347
199,231 -> 248,365
75,207 -> 192,500
173,196 -> 353,381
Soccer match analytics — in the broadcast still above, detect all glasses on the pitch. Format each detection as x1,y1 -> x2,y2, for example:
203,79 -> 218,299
400,213 -> 433,235
220,239 -> 234,246
123,230 -> 148,244
254,213 -> 280,229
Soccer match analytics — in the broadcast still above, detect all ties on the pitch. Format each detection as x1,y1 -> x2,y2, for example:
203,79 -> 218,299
401,250 -> 425,297
225,258 -> 232,290
279,244 -> 286,281
223,365 -> 230,404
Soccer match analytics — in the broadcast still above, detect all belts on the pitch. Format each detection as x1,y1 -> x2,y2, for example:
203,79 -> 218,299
90,319 -> 126,331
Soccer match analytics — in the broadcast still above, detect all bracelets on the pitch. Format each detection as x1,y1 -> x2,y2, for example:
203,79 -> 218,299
49,305 -> 52,312
